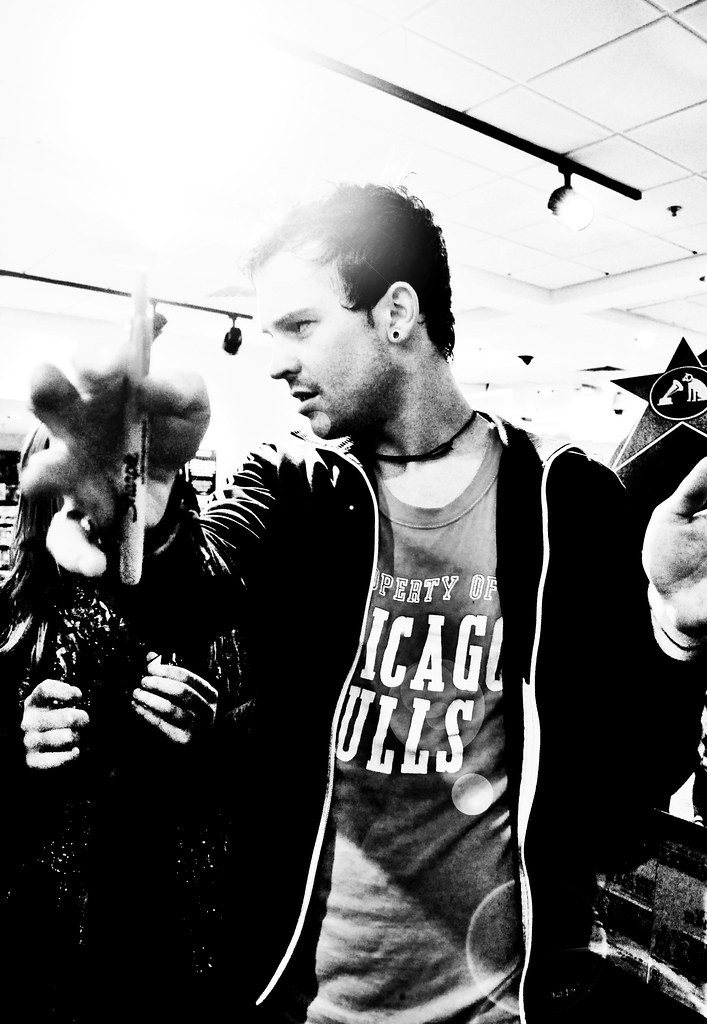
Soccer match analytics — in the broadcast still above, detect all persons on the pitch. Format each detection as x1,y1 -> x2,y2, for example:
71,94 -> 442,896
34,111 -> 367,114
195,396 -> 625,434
0,180 -> 707,1024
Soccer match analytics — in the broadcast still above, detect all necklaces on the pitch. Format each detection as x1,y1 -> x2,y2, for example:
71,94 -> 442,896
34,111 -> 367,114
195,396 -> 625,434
376,410 -> 478,461
